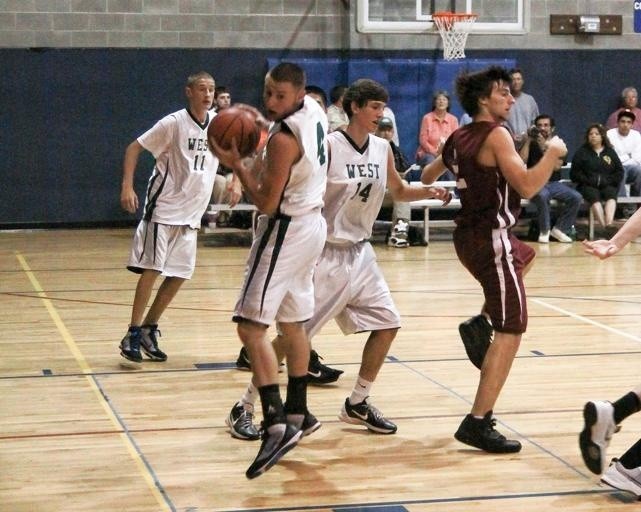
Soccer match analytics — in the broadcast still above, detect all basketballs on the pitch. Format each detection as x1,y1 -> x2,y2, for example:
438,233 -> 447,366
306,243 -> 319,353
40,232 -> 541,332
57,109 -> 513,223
208,107 -> 260,160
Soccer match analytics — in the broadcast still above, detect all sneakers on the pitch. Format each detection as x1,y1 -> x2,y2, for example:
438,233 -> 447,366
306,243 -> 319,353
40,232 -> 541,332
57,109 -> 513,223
459,315 -> 493,369
580,400 -> 641,497
539,226 -> 573,243
454,410 -> 522,452
225,347 -> 397,479
387,218 -> 428,248
119,324 -> 167,361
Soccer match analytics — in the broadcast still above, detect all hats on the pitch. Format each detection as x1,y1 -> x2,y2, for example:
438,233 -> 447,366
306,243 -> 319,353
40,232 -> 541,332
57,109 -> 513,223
377,117 -> 393,128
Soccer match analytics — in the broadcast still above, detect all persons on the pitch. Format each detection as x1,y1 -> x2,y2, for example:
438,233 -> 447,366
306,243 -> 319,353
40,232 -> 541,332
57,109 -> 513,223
117,62 -> 641,502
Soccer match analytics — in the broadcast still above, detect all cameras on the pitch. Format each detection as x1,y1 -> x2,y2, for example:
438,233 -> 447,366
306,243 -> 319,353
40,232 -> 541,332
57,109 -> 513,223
529,127 -> 547,137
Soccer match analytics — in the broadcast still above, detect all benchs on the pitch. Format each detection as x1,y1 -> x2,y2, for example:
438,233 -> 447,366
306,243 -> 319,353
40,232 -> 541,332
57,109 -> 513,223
203,156 -> 641,248
